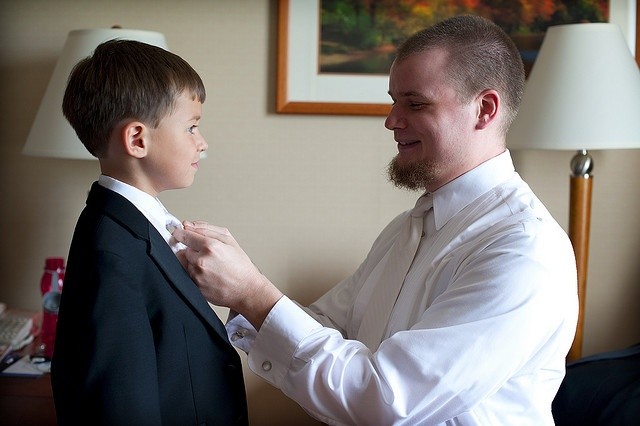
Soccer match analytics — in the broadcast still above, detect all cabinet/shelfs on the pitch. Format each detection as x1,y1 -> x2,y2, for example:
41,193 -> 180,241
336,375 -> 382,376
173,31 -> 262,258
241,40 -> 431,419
0,331 -> 56,426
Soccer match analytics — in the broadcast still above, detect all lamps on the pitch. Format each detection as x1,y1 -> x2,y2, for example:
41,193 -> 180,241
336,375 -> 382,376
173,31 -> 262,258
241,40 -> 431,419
21,23 -> 171,160
504,20 -> 640,363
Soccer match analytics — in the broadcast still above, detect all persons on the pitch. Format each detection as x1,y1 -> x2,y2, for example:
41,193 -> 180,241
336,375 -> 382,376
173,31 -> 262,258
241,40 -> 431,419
173,14 -> 578,425
51,40 -> 249,426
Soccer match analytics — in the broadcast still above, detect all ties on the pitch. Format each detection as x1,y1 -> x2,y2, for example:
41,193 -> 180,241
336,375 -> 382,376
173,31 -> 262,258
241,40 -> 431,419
165,211 -> 185,231
352,195 -> 433,354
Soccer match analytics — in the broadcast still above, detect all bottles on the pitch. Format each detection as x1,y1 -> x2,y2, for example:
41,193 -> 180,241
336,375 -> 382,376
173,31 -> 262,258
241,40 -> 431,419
40,257 -> 65,360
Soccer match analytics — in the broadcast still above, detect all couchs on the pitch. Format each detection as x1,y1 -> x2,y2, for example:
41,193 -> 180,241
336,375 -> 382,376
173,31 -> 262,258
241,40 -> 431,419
549,344 -> 639,425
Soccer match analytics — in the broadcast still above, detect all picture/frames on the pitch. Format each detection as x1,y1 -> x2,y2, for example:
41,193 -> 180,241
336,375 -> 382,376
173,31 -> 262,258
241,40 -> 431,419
275,0 -> 640,115
